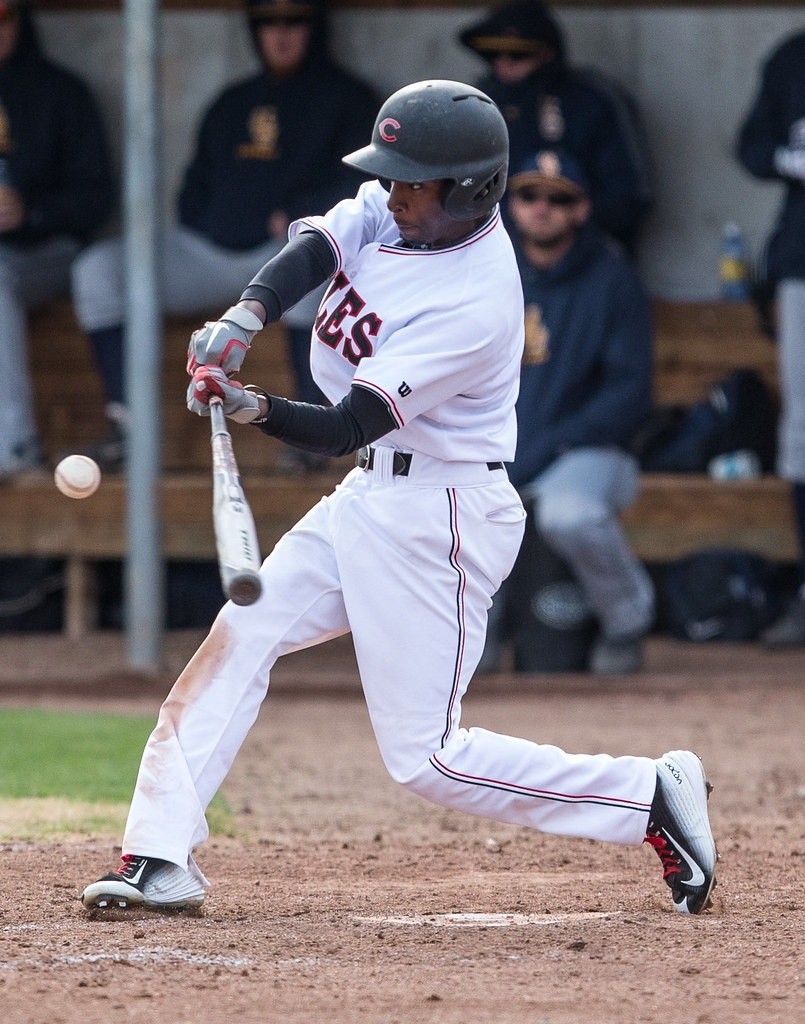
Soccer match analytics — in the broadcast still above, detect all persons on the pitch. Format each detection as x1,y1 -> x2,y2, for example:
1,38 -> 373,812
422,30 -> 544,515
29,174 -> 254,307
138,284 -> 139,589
80,80 -> 717,915
734,28 -> 805,479
0,0 -> 110,471
71,0 -> 388,474
457,0 -> 659,267
503,144 -> 654,676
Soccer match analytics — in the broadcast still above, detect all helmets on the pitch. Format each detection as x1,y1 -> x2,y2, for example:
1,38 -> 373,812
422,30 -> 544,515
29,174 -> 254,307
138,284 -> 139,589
342,80 -> 509,222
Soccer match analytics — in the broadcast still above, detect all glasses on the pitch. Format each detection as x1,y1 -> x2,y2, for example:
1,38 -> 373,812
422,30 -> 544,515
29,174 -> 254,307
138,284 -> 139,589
482,49 -> 536,61
509,188 -> 581,207
254,15 -> 303,27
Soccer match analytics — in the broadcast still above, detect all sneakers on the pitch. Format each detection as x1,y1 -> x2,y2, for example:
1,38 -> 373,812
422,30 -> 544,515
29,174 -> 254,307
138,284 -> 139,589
82,854 -> 205,909
643,750 -> 717,913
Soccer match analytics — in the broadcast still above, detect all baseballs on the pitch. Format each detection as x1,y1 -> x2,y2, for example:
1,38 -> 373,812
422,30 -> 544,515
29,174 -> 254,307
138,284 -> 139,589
54,454 -> 102,500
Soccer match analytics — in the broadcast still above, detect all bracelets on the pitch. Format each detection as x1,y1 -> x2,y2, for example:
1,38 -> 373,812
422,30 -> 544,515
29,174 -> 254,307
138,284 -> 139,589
243,383 -> 274,425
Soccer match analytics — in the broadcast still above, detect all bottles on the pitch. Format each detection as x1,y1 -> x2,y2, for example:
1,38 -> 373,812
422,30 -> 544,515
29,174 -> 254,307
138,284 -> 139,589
716,220 -> 756,300
711,451 -> 758,482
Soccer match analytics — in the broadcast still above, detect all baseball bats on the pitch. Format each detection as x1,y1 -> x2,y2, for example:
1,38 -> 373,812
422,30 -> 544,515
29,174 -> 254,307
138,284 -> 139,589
208,395 -> 264,606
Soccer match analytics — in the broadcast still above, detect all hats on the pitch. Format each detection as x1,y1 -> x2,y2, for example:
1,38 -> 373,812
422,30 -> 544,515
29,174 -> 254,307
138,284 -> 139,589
509,147 -> 590,192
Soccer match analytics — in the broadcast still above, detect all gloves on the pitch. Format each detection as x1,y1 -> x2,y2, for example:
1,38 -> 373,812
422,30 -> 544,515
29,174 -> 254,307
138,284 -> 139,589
185,307 -> 264,378
186,365 -> 260,424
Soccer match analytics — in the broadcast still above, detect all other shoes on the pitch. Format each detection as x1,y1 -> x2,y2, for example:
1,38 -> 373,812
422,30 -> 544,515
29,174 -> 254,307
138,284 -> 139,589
590,633 -> 643,672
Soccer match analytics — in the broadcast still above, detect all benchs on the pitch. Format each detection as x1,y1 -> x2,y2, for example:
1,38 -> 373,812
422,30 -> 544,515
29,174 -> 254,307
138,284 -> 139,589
1,285 -> 805,642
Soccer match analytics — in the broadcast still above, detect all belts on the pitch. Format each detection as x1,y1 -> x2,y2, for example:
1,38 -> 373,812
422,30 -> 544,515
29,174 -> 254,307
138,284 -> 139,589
356,445 -> 506,476
